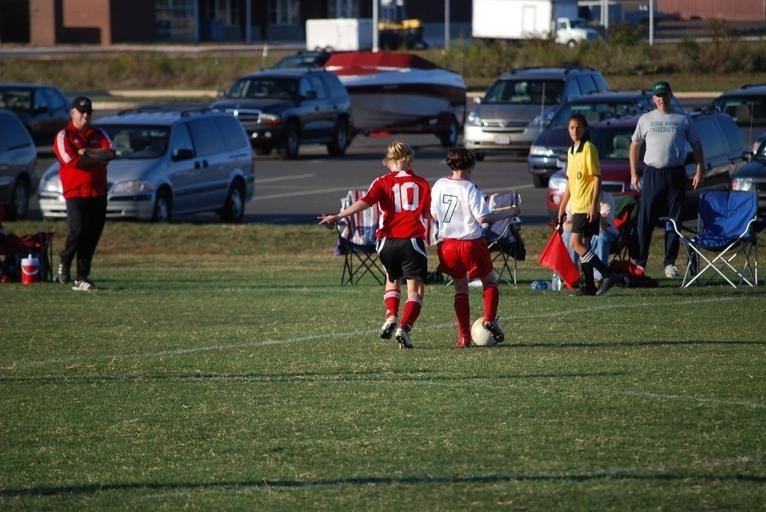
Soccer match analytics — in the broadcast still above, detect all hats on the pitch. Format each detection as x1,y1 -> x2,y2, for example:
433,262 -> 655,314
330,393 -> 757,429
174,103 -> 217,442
72,96 -> 95,113
650,81 -> 671,95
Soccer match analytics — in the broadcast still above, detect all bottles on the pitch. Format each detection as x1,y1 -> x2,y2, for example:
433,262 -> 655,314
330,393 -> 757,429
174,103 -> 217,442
530,281 -> 552,289
552,269 -> 562,291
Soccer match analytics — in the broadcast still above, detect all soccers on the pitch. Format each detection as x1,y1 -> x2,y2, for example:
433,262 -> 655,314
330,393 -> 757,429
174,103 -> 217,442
469,316 -> 498,347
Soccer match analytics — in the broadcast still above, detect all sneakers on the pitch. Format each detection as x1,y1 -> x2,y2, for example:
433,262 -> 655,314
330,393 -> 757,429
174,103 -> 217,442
482,318 -> 504,343
455,334 -> 471,347
664,265 -> 681,278
576,286 -> 598,296
596,272 -> 618,296
72,278 -> 97,292
378,315 -> 397,340
395,328 -> 415,349
58,262 -> 71,284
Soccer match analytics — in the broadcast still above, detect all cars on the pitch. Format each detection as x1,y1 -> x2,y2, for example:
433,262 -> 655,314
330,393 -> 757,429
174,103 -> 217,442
727,133 -> 766,217
0,85 -> 75,145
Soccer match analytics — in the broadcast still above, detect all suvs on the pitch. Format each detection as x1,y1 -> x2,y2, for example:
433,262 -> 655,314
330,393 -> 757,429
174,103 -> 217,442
206,66 -> 353,159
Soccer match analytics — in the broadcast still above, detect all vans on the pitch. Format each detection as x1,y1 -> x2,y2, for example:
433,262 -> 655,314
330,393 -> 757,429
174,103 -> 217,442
37,107 -> 255,223
533,88 -> 685,188
545,109 -> 749,231
1,109 -> 41,220
462,66 -> 609,162
712,84 -> 765,161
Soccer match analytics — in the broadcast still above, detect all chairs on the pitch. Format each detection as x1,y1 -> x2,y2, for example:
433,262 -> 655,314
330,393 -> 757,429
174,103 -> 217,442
333,190 -> 388,285
659,188 -> 758,290
443,190 -> 521,287
611,196 -> 635,264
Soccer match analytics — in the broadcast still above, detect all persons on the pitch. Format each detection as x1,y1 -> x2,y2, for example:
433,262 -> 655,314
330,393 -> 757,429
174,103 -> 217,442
54,96 -> 115,293
558,114 -> 616,296
630,80 -> 703,279
318,142 -> 432,348
431,147 -> 521,346
561,192 -> 620,288
